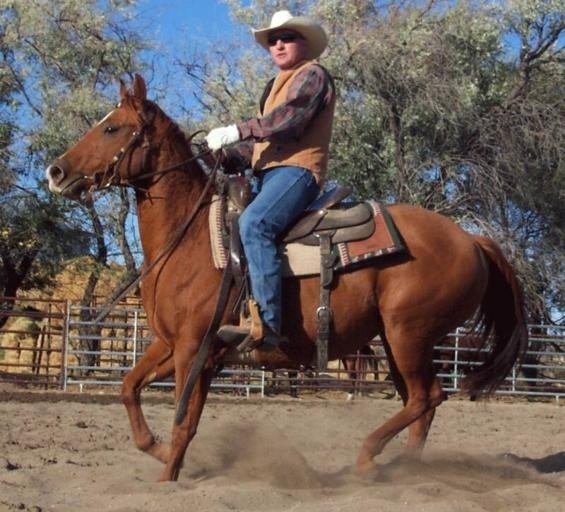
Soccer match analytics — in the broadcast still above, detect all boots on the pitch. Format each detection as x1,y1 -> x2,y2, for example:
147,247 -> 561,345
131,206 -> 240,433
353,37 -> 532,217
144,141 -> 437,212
215,316 -> 278,353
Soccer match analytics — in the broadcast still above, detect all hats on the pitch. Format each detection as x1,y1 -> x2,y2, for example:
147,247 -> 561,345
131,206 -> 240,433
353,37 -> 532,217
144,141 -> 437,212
249,9 -> 328,61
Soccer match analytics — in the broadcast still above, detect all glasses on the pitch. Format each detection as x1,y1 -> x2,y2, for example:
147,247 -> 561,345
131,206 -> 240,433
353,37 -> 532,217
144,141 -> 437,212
264,32 -> 302,45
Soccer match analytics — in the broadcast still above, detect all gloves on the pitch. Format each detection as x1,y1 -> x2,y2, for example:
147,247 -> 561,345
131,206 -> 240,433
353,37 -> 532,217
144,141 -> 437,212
202,123 -> 240,153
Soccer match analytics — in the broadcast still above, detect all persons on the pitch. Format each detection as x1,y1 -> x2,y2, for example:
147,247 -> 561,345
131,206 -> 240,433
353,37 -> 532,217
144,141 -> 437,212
203,8 -> 335,354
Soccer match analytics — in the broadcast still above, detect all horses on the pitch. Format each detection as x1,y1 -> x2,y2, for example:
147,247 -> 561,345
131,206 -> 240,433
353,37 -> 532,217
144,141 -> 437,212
44,71 -> 529,487
343,329 -> 565,403
